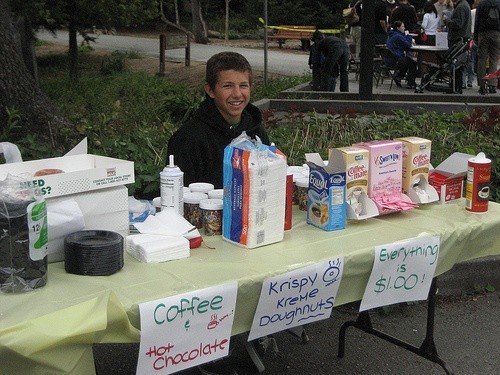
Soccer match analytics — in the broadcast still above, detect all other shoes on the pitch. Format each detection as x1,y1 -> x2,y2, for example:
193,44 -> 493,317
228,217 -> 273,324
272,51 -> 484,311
490,86 -> 497,94
407,84 -> 416,89
479,86 -> 487,95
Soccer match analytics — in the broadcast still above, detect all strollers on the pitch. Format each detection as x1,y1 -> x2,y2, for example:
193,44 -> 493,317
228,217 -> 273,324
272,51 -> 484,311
414,35 -> 476,94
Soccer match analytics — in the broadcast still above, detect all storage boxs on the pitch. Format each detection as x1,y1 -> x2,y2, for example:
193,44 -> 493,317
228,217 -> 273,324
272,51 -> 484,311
351,140 -> 414,216
285,175 -> 293,230
394,136 -> 439,204
305,150 -> 347,232
48,185 -> 129,263
465,152 -> 492,213
429,152 -> 476,204
0,154 -> 135,202
329,146 -> 379,220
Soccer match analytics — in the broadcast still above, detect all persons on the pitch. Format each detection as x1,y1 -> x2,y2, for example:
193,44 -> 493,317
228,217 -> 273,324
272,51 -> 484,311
308,0 -> 500,96
165,52 -> 270,189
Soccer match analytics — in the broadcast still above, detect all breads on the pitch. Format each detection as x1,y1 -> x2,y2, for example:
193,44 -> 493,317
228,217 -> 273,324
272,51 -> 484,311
34,168 -> 64,176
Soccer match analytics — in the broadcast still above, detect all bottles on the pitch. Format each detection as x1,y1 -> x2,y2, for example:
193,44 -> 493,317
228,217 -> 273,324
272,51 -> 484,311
159,155 -> 184,216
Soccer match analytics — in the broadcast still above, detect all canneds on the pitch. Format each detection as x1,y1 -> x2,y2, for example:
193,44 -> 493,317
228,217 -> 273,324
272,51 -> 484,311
464,152 -> 491,213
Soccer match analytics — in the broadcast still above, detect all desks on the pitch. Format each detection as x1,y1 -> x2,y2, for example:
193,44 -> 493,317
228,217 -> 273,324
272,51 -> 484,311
0,196 -> 500,375
410,45 -> 449,88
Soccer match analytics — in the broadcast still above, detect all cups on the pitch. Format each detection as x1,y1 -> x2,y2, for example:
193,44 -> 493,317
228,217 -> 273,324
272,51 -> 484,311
287,164 -> 309,211
183,183 -> 223,236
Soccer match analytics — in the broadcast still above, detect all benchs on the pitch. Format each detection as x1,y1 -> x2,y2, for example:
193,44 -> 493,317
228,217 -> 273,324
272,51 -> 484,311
266,25 -> 317,51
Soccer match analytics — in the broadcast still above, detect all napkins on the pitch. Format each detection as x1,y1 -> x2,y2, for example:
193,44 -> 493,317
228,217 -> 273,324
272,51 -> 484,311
125,208 -> 201,264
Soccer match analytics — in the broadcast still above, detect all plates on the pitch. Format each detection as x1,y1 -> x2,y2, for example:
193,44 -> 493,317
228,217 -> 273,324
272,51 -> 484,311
64,230 -> 124,276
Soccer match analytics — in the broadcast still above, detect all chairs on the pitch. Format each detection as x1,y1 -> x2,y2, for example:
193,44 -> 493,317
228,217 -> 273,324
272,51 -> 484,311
414,36 -> 478,94
351,45 -> 386,85
376,47 -> 412,90
